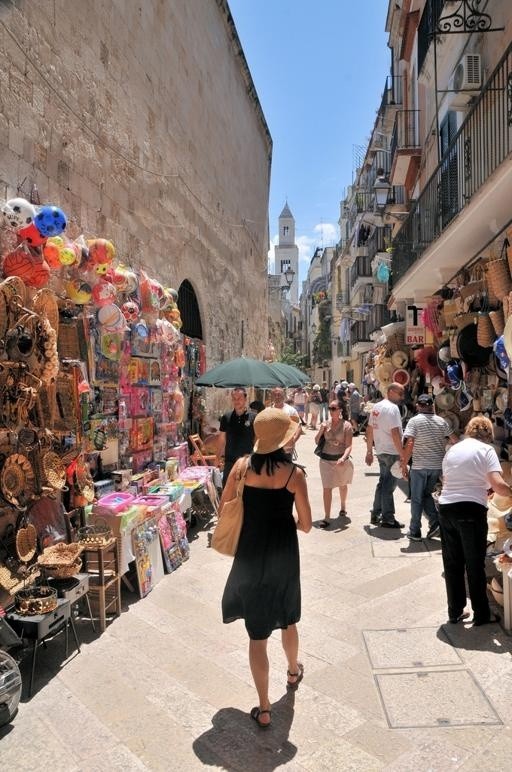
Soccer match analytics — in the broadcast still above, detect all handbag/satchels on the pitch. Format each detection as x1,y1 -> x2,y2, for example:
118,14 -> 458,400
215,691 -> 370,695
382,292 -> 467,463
210,499 -> 244,556
443,228 -> 512,359
314,435 -> 325,457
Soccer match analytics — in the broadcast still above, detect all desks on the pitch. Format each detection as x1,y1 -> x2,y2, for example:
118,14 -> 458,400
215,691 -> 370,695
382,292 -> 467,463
84,465 -> 217,593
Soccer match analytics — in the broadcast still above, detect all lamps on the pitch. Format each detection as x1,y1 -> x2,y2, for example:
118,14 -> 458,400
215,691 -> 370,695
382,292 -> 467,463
371,172 -> 409,222
268,266 -> 295,291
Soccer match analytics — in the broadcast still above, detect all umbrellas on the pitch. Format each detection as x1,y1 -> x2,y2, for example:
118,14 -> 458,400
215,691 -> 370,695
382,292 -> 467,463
270,360 -> 304,390
193,353 -> 286,392
290,364 -> 312,381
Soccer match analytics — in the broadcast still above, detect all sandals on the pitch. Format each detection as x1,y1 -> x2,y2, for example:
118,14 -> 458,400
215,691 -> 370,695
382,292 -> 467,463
251,707 -> 272,728
286,663 -> 303,690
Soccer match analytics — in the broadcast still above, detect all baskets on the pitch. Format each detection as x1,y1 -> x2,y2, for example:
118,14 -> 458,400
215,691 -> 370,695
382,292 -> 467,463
31,288 -> 58,337
1,274 -> 26,337
0,452 -> 113,578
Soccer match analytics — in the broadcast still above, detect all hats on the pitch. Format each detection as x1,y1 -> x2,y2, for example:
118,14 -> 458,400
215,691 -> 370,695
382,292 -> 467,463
253,406 -> 299,455
417,394 -> 432,407
486,460 -> 512,574
431,348 -> 472,432
375,349 -> 411,386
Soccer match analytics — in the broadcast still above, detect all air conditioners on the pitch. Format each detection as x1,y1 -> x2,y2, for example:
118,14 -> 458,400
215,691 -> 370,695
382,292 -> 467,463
447,53 -> 483,112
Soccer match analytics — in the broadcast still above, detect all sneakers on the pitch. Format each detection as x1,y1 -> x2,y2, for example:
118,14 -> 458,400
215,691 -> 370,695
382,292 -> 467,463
450,611 -> 500,625
371,516 -> 440,542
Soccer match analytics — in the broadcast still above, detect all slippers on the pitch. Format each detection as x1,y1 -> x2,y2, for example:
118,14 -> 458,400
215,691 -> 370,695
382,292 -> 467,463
339,510 -> 347,516
320,520 -> 330,528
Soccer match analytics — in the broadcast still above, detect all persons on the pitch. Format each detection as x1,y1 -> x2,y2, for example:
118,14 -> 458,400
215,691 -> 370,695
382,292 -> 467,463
219,376 -> 462,543
437,414 -> 512,628
216,405 -> 315,729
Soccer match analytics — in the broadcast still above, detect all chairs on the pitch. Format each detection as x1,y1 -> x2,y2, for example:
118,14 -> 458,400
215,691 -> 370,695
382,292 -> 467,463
188,433 -> 218,466
63,505 -> 121,632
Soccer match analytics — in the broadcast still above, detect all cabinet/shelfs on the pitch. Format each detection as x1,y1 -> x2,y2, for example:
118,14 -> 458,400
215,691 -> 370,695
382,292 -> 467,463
35,573 -> 96,654
4,599 -> 70,697
